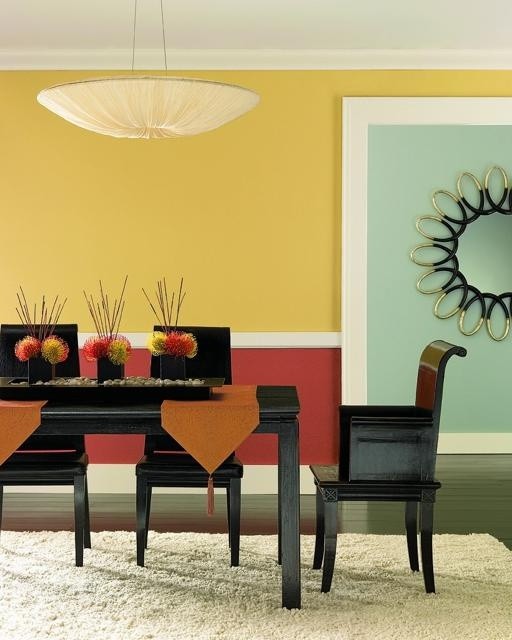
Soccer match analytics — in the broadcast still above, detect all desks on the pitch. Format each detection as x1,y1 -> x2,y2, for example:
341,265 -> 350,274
1,376 -> 302,613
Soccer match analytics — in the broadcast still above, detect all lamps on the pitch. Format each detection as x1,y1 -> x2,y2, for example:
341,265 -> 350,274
37,0 -> 267,148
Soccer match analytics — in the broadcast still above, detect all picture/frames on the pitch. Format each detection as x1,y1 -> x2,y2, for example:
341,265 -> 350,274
338,93 -> 512,456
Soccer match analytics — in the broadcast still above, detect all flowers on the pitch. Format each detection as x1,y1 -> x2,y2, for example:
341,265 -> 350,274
81,275 -> 133,366
142,277 -> 198,358
13,286 -> 72,364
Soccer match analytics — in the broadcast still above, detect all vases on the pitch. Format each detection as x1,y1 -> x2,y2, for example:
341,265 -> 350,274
97,356 -> 125,383
159,355 -> 187,381
27,357 -> 55,385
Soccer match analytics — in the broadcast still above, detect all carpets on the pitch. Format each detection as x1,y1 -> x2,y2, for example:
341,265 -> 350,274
0,527 -> 512,640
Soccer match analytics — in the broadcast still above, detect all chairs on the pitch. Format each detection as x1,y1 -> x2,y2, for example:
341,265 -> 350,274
310,341 -> 467,594
0,323 -> 91,569
135,325 -> 242,568
